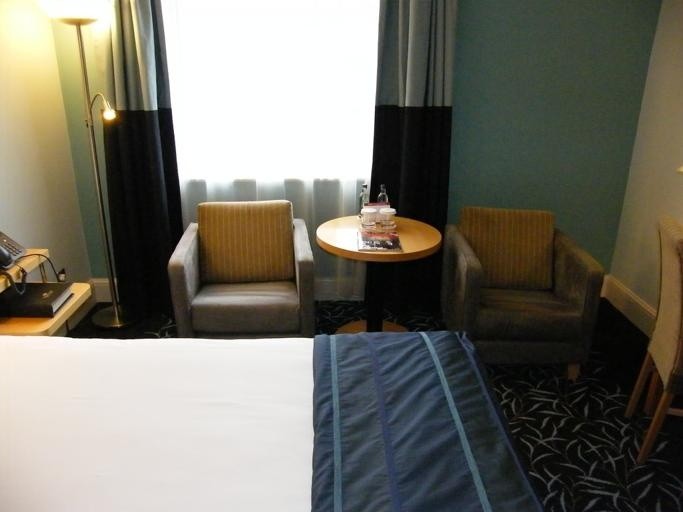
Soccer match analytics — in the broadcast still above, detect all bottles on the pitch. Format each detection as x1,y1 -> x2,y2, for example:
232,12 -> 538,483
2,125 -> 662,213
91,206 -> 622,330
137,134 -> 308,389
377,183 -> 388,202
359,183 -> 372,218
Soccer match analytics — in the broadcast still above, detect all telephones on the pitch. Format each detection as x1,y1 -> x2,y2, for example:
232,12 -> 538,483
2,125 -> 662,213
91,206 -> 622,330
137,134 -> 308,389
0,231 -> 27,270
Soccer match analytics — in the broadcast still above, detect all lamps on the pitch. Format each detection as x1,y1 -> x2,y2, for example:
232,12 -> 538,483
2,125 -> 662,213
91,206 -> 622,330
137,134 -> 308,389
43,0 -> 145,335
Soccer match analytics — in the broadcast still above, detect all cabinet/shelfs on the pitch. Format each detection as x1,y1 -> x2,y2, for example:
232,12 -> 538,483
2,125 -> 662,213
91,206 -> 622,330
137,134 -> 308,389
0,248 -> 92,335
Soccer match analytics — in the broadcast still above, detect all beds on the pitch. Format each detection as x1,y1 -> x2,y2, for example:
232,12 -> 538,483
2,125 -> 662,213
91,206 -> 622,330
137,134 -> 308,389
0,331 -> 545,512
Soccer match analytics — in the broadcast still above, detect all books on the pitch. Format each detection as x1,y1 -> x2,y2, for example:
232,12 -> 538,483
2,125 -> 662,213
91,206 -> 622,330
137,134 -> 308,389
0,283 -> 75,318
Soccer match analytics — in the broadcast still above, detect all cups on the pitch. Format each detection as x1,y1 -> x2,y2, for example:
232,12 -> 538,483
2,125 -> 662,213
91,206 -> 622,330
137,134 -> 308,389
379,207 -> 397,229
362,209 -> 376,229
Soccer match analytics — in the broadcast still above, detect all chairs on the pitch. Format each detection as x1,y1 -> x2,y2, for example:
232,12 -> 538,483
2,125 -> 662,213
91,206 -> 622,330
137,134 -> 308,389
445,206 -> 605,382
624,213 -> 683,464
168,200 -> 315,338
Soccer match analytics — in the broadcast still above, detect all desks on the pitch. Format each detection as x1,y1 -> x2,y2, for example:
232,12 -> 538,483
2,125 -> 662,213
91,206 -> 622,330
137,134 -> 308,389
315,214 -> 442,335
